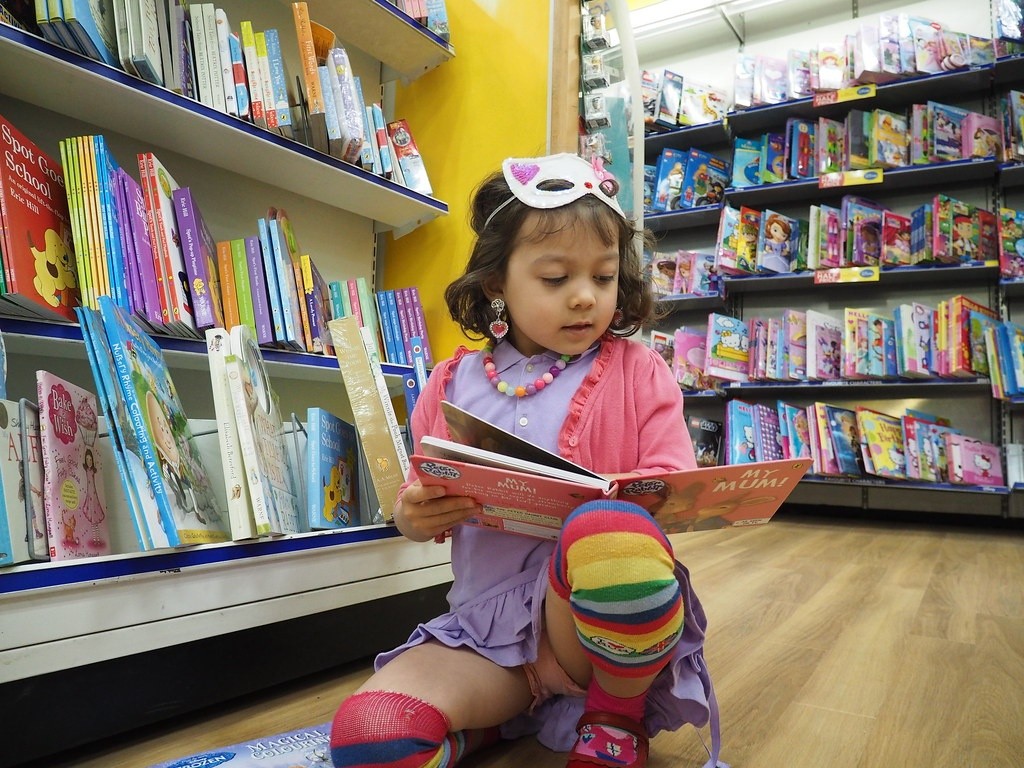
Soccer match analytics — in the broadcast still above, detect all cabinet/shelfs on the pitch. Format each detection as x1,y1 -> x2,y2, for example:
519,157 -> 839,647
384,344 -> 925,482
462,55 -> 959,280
0,0 -> 644,765
640,50 -> 1024,523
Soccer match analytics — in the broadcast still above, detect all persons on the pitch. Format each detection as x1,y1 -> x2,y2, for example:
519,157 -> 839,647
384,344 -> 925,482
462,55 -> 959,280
329,153 -> 720,768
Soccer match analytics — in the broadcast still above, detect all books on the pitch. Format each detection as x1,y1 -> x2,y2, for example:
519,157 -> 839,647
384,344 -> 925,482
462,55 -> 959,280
409,399 -> 814,539
0,0 -> 449,569
633,0 -> 1024,493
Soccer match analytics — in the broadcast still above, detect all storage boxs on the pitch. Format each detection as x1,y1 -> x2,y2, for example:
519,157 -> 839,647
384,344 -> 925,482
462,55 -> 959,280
583,12 -> 613,166
644,89 -> 1024,216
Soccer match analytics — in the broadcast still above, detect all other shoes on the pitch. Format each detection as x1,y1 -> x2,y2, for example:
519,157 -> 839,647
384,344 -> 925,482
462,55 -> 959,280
566,711 -> 650,768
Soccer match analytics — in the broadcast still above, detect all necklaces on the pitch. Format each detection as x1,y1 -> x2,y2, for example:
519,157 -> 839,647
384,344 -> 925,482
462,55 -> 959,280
482,337 -> 573,397
